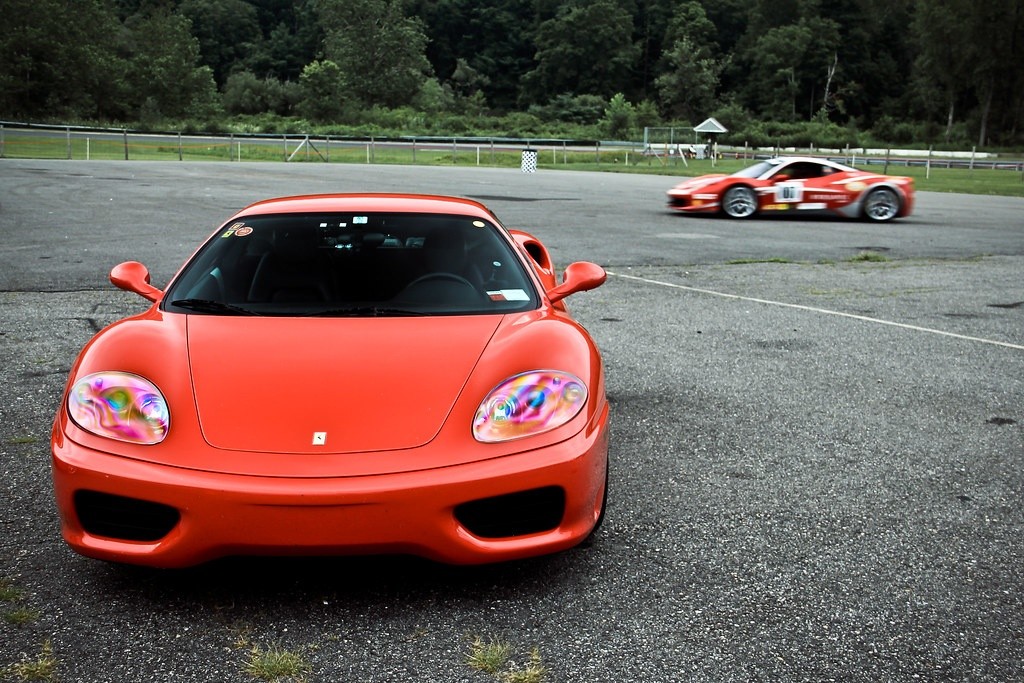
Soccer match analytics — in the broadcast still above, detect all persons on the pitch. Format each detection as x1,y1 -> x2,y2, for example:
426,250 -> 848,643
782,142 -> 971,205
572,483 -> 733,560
688,146 -> 708,159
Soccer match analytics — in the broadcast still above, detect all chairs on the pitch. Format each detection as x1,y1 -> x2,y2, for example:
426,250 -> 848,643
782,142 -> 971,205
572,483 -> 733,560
249,228 -> 320,304
405,228 -> 482,307
355,233 -> 384,251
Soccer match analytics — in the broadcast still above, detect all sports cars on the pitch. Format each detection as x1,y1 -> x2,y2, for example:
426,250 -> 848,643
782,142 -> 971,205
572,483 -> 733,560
50,196 -> 610,569
666,156 -> 916,219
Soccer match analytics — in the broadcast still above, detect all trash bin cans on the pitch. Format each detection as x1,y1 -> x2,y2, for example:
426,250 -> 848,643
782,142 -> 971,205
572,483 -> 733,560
521,149 -> 538,173
